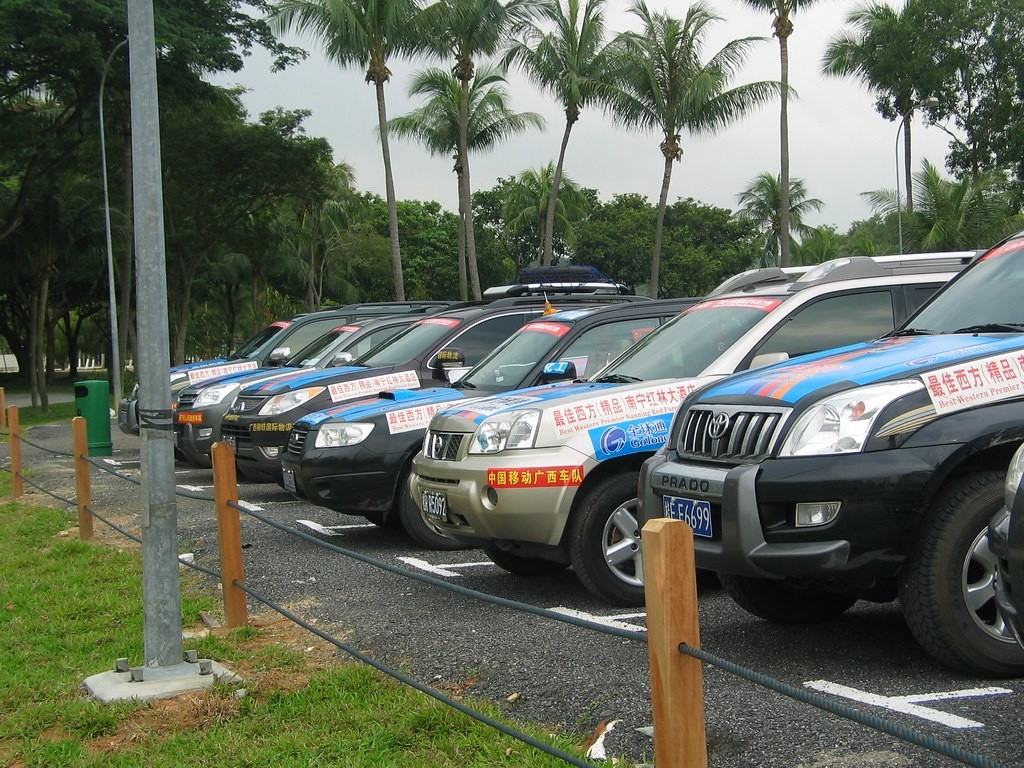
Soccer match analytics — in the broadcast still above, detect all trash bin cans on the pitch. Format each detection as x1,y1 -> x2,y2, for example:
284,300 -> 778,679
73,380 -> 112,456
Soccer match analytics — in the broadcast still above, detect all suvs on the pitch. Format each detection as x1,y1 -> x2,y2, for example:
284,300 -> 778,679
405,245 -> 994,611
221,261 -> 632,485
638,228 -> 1024,676
116,296 -> 465,472
273,294 -> 705,549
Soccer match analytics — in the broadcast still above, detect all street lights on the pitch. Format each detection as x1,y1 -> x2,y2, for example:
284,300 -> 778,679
894,96 -> 943,253
